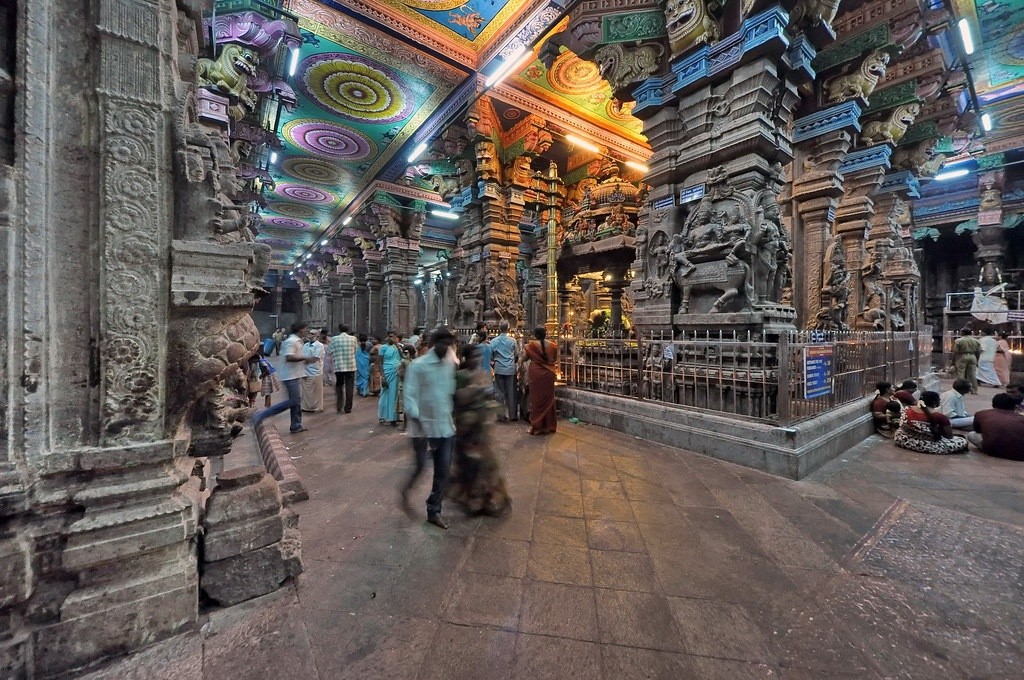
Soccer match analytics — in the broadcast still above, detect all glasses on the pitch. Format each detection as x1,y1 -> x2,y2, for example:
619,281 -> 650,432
403,352 -> 408,355
309,333 -> 314,335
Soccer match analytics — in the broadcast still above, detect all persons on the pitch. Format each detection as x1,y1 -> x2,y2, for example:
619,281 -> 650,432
214,150 -> 272,278
978,325 -> 1011,388
870,378 -> 975,455
461,273 -> 479,294
967,381 -> 1024,461
949,327 -> 980,394
402,321 -> 519,529
754,202 -> 789,303
555,206 -> 627,248
634,226 -> 672,280
521,326 -> 558,436
672,206 -> 750,276
252,322 -> 325,433
355,330 -> 401,425
273,327 -> 285,356
491,280 -> 529,320
251,353 -> 281,408
328,323 -> 358,413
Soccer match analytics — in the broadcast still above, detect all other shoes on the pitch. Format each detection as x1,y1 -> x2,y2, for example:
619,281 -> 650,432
992,384 -> 999,388
427,509 -> 450,529
345,410 -> 350,413
399,423 -> 407,431
391,421 -> 398,426
978,382 -> 981,386
290,426 -> 309,434
510,417 -> 518,421
